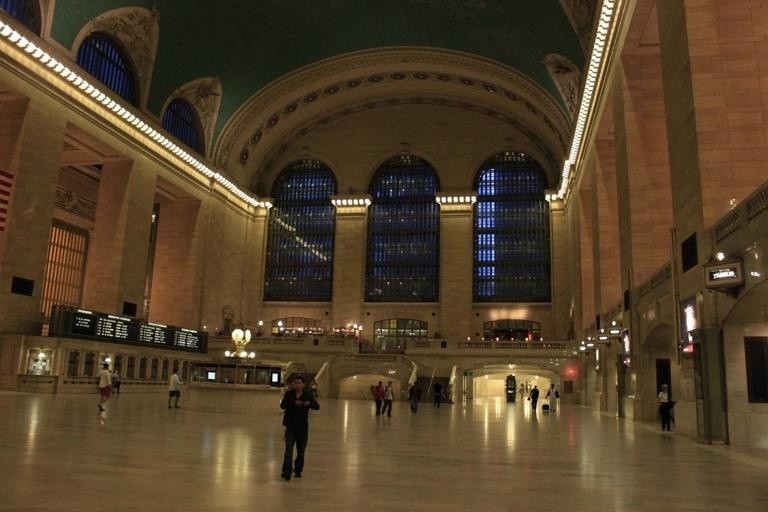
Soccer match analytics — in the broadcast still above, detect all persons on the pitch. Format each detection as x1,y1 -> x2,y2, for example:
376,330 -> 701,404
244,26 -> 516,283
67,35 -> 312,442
280,375 -> 320,480
546,383 -> 560,412
381,380 -> 396,417
527,384 -> 539,410
658,383 -> 672,431
408,380 -> 424,416
112,369 -> 121,393
517,381 -> 525,400
167,367 -> 185,407
373,380 -> 384,415
95,364 -> 112,411
432,380 -> 443,409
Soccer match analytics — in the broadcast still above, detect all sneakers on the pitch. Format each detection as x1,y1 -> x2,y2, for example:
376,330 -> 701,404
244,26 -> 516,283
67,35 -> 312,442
281,472 -> 291,480
168,405 -> 181,410
97,404 -> 106,411
294,471 -> 303,478
